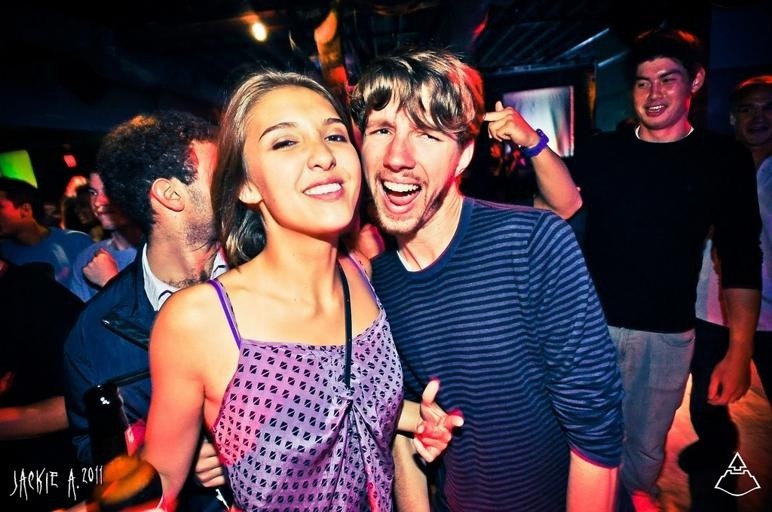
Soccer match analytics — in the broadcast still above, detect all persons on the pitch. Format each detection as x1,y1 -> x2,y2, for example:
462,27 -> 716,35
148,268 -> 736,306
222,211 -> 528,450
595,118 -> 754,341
0,29 -> 772,509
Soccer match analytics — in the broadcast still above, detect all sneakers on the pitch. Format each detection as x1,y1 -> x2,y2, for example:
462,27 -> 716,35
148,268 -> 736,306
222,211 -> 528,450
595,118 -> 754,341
680,424 -> 739,462
